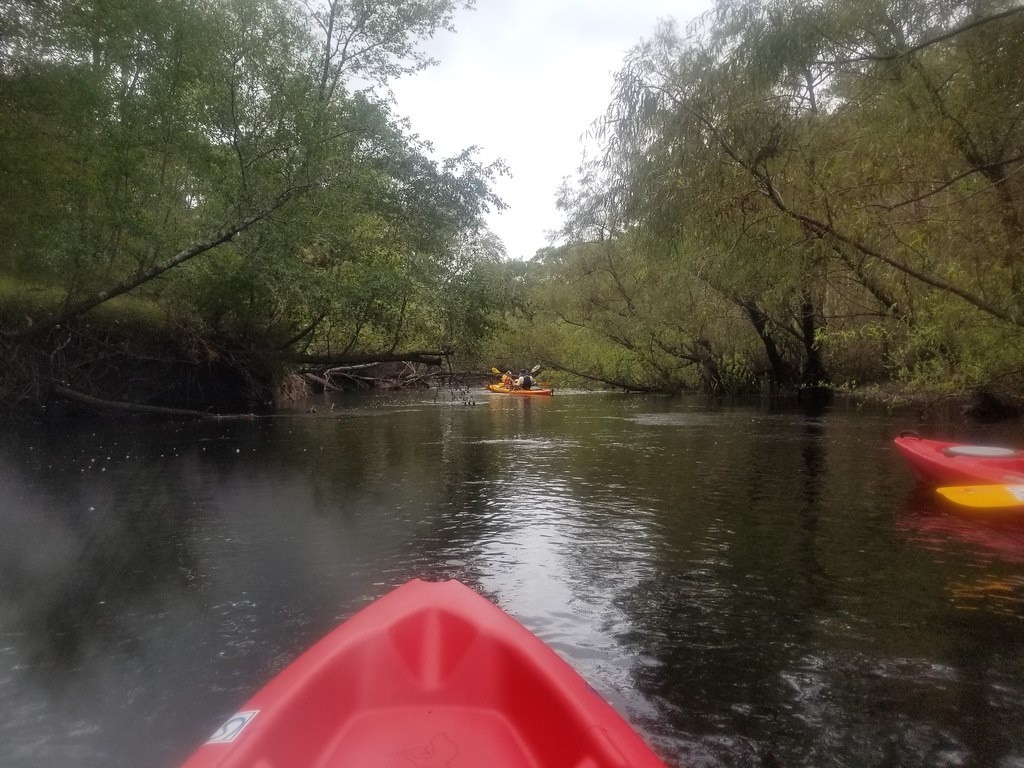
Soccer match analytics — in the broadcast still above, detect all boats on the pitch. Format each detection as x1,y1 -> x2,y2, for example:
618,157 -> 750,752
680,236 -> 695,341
486,384 -> 552,396
894,429 -> 1024,525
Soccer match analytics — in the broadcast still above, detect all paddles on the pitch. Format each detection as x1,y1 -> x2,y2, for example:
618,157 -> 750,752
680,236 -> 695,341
492,367 -> 504,376
528,364 -> 540,375
937,483 -> 1024,508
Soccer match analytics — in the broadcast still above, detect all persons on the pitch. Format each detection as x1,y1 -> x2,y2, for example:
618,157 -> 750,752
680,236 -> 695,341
498,371 -> 513,387
514,369 -> 535,390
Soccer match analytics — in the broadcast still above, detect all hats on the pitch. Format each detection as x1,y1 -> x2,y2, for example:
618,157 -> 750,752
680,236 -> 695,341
505,371 -> 512,374
519,369 -> 528,376
